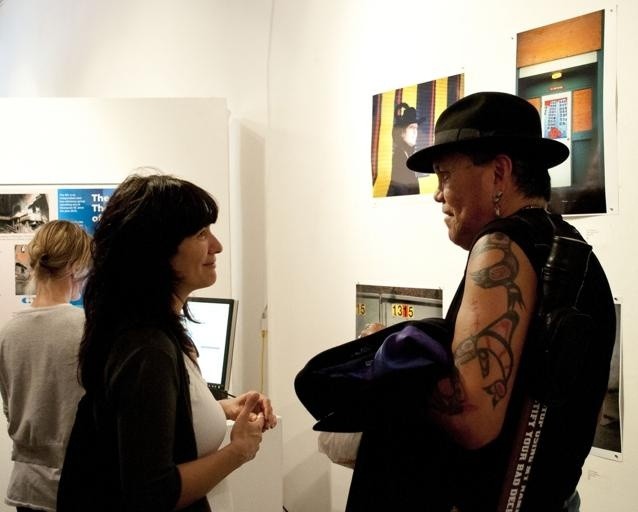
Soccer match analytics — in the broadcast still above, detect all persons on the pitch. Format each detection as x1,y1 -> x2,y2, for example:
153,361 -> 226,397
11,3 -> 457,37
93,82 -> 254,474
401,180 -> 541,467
56,174 -> 277,512
387,106 -> 427,197
317,90 -> 617,512
0,221 -> 94,512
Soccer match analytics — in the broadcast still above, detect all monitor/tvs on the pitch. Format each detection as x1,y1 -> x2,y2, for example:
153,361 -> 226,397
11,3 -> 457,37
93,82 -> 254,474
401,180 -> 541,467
178,296 -> 239,400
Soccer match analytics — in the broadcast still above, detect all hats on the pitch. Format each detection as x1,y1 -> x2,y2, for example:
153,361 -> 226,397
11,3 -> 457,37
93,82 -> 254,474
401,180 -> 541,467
394,107 -> 426,127
406,91 -> 570,174
